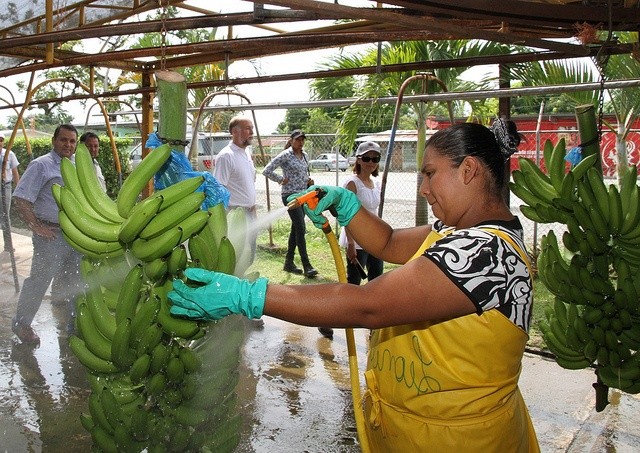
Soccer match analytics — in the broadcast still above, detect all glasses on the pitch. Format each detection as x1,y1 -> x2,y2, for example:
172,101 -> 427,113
358,156 -> 381,164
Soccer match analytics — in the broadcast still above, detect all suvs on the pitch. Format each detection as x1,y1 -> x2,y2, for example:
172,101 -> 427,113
129,132 -> 232,172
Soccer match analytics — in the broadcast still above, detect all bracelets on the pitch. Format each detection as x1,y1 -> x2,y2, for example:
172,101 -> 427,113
25,219 -> 44,230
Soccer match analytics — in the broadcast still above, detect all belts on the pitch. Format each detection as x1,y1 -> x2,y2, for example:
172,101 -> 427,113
38,218 -> 61,228
5,181 -> 13,184
229,205 -> 256,213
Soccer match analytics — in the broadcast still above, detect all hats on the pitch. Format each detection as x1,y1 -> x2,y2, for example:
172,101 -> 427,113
356,141 -> 382,159
0,132 -> 5,139
291,131 -> 307,139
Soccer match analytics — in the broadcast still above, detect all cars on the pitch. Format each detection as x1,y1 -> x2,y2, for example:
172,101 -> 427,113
309,153 -> 349,172
348,156 -> 357,166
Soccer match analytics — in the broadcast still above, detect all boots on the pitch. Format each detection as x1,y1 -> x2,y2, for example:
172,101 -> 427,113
301,256 -> 318,277
284,253 -> 304,274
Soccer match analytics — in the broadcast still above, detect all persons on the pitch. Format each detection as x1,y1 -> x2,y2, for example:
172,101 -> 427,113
79,133 -> 108,193
263,129 -> 318,278
1,135 -> 19,252
319,141 -> 383,337
168,115 -> 539,451
11,123 -> 79,346
214,118 -> 258,275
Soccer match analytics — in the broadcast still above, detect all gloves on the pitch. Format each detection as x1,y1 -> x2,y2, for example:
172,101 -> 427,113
166,268 -> 270,320
286,186 -> 365,229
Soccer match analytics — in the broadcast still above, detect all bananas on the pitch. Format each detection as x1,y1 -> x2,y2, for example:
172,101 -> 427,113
53,139 -> 264,453
508,137 -> 639,393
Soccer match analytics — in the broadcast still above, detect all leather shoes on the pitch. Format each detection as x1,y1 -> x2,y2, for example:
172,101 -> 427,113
5,248 -> 16,252
252,318 -> 264,327
318,327 -> 334,337
12,318 -> 41,344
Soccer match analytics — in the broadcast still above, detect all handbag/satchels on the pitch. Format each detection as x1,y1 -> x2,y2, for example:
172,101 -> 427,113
302,152 -> 315,189
